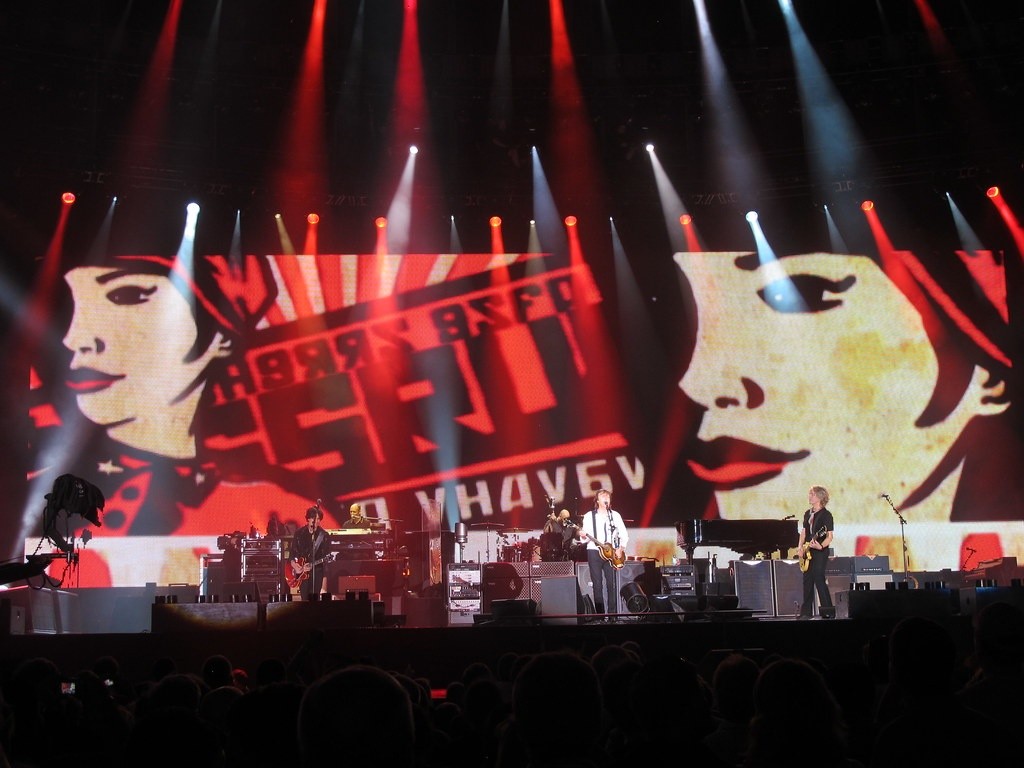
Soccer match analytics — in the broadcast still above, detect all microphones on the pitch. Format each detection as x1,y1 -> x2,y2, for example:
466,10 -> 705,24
351,515 -> 358,518
879,493 -> 890,498
317,498 -> 321,506
605,501 -> 609,509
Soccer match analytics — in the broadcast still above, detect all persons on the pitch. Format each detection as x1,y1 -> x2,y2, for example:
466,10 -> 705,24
342,503 -> 371,529
286,521 -> 297,534
579,489 -> 628,623
289,506 -> 339,601
0,601 -> 1024,768
543,509 -> 574,550
796,486 -> 835,620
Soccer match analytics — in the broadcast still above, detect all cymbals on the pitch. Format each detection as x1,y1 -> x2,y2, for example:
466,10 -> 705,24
471,522 -> 505,527
499,527 -> 533,533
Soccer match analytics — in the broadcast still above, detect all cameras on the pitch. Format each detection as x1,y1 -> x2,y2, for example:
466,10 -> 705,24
57,678 -> 79,697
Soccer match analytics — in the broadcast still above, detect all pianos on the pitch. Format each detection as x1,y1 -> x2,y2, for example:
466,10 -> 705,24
675,514 -> 801,565
324,528 -> 390,541
964,557 -> 1018,587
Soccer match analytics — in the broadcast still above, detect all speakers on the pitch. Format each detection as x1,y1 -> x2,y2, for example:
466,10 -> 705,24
0,585 -> 80,635
729,560 -> 816,617
481,561 -> 656,626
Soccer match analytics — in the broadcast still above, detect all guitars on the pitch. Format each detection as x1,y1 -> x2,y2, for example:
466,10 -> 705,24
285,552 -> 336,589
563,517 -> 626,570
798,525 -> 828,573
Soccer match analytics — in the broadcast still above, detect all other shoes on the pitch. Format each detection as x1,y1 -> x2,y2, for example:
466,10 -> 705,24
609,616 -> 618,623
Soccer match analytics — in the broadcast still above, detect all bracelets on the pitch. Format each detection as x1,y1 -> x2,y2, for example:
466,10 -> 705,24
821,545 -> 823,549
